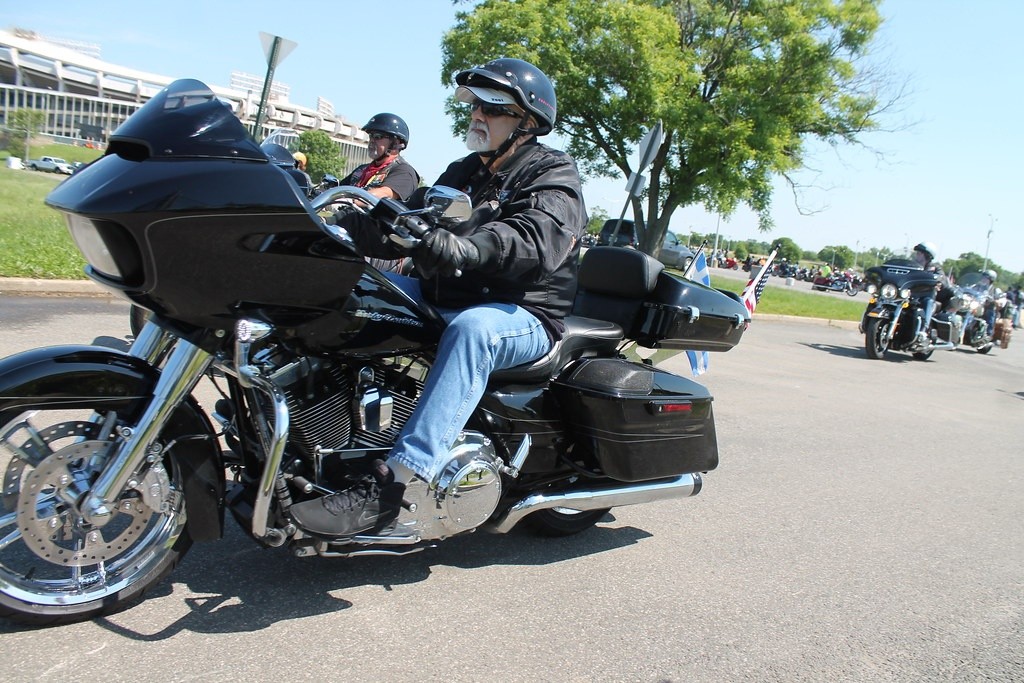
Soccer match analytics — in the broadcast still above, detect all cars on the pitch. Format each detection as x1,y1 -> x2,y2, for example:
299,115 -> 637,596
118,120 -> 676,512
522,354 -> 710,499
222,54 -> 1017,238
595,219 -> 694,271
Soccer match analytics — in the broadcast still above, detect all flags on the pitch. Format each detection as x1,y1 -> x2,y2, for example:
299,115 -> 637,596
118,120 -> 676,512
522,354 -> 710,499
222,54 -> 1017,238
685,251 -> 710,376
742,260 -> 773,331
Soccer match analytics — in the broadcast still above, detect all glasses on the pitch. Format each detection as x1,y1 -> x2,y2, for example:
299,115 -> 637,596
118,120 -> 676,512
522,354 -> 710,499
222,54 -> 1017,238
369,131 -> 393,139
470,100 -> 521,121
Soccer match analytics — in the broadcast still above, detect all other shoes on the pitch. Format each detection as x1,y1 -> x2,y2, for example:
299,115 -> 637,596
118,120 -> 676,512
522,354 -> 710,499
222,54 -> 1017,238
917,335 -> 925,342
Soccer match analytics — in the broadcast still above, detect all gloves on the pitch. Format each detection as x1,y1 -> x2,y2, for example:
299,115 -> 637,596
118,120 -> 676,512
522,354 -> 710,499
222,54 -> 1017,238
411,227 -> 478,281
324,195 -> 354,213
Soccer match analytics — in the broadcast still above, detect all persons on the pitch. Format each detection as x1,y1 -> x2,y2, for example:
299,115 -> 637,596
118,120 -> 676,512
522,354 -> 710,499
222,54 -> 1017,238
746,254 -> 766,265
324,113 -> 420,213
913,241 -> 945,343
288,57 -> 589,540
294,151 -> 315,189
978,269 -> 1007,340
1005,285 -> 1024,328
780,259 -> 831,277
583,230 -> 601,245
718,249 -> 728,262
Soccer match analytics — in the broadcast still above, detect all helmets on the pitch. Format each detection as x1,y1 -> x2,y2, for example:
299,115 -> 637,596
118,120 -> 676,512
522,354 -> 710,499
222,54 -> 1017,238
983,269 -> 997,285
915,243 -> 935,261
361,112 -> 409,149
459,58 -> 556,136
292,152 -> 307,166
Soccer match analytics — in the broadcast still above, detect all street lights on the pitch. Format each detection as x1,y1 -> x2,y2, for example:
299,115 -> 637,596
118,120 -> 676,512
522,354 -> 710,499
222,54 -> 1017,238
983,213 -> 998,273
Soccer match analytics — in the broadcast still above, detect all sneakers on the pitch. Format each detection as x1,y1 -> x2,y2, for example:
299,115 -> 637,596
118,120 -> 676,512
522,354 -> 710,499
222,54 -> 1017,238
290,458 -> 406,538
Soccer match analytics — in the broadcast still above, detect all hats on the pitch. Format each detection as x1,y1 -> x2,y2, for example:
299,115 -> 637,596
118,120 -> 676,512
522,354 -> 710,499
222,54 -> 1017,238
454,83 -> 541,128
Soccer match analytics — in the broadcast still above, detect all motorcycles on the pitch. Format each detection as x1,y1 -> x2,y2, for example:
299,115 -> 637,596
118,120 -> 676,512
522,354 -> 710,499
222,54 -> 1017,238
0,79 -> 753,629
814,268 -> 858,297
860,248 -> 963,361
954,273 -> 1007,354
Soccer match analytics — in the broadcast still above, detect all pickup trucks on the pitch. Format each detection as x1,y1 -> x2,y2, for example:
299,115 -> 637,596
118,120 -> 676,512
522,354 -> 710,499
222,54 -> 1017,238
26,155 -> 77,174
